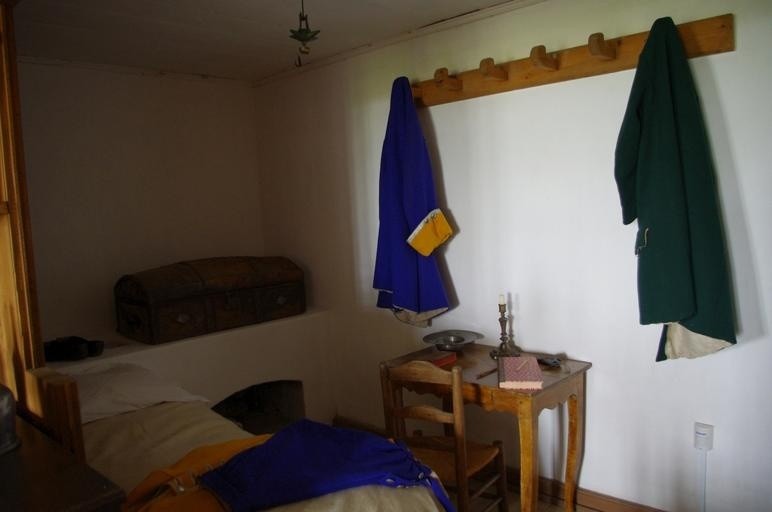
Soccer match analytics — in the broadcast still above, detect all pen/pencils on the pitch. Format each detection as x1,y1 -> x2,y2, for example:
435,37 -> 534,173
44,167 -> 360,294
515,358 -> 528,373
475,366 -> 501,381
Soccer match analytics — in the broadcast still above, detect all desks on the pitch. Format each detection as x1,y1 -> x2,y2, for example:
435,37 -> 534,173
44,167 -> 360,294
378,342 -> 592,512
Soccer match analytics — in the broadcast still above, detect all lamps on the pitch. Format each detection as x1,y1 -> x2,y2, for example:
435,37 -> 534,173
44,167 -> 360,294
280,0 -> 322,90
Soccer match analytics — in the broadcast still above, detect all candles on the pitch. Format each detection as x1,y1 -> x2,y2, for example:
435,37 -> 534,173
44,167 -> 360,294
498,294 -> 505,304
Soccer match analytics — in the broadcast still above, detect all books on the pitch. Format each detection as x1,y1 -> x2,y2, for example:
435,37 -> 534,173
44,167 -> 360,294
497,354 -> 545,392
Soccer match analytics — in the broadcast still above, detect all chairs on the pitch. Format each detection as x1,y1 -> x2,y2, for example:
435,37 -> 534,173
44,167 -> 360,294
378,359 -> 507,512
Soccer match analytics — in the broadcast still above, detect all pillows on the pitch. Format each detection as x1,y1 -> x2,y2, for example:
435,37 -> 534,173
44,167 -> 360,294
72,362 -> 269,512
